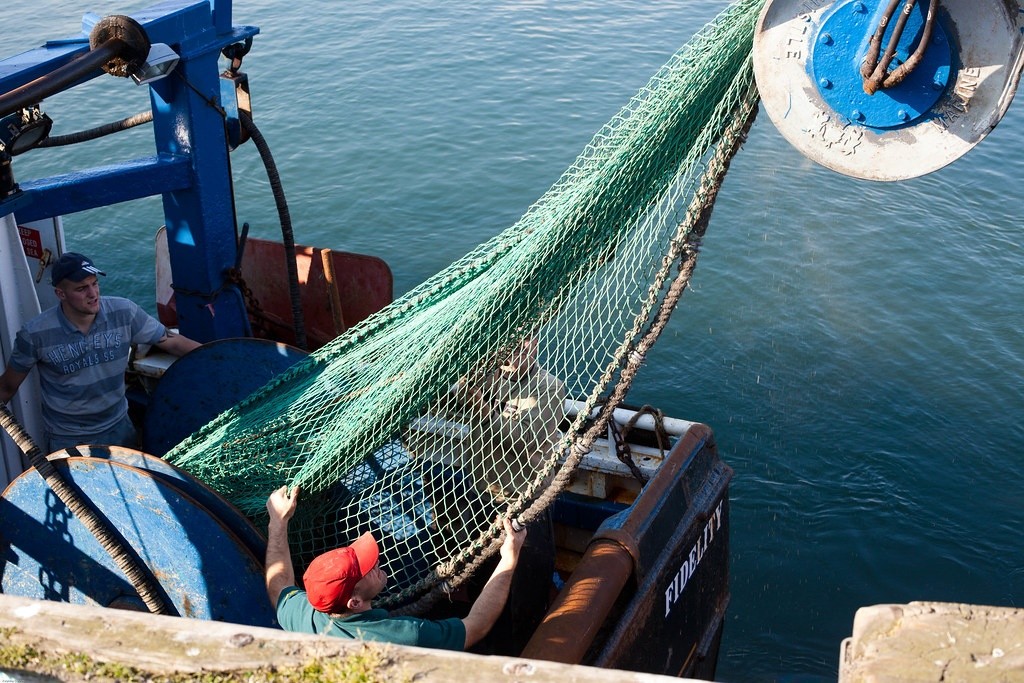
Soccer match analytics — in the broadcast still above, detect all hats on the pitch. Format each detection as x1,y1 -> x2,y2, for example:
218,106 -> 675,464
51,252 -> 106,286
302,531 -> 379,613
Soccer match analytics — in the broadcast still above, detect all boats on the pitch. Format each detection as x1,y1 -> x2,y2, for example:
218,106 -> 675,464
0,0 -> 1024,683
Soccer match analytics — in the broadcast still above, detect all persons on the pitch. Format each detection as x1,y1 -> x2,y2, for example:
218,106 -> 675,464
264,485 -> 527,651
0,253 -> 203,453
452,323 -> 564,570
